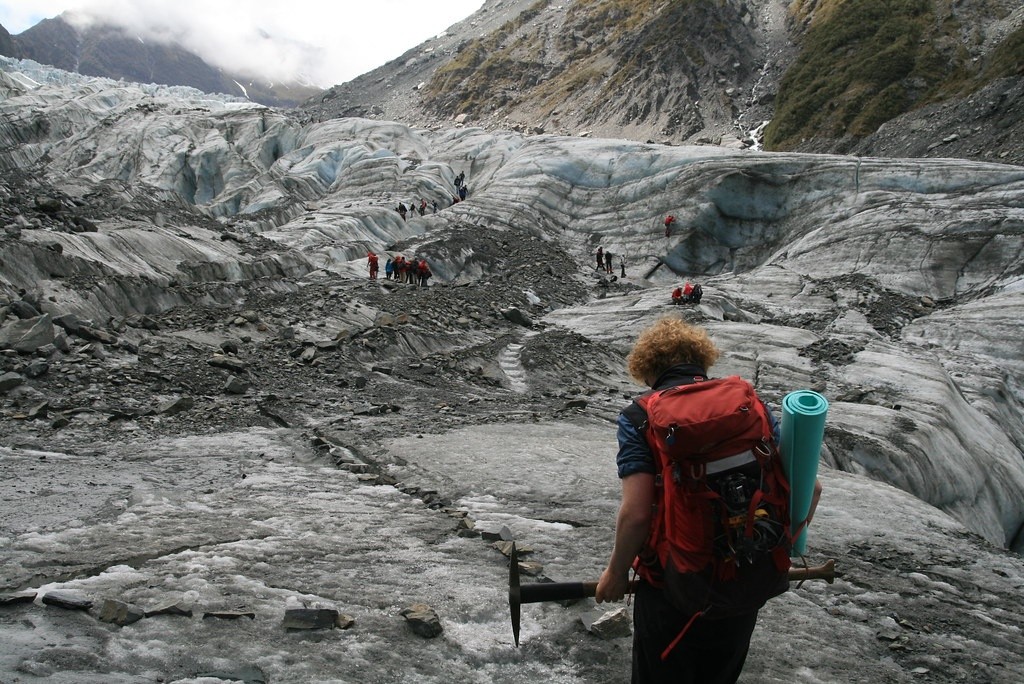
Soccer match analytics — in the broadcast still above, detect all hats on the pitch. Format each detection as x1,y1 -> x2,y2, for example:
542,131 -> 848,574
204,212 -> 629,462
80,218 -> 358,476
679,285 -> 683,291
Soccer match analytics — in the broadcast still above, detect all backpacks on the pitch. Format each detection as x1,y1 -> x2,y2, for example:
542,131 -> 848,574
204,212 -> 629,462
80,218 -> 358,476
636,371 -> 796,657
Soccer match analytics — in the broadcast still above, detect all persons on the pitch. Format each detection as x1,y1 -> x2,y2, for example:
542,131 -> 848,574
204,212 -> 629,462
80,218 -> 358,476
367,250 -> 432,287
459,170 -> 466,186
672,282 -> 703,306
409,202 -> 416,218
453,176 -> 461,194
399,202 -> 407,222
595,314 -> 824,684
621,254 -> 627,278
464,185 -> 468,200
459,187 -> 466,200
595,246 -> 614,274
420,198 -> 425,216
432,199 -> 439,213
664,214 -> 675,238
